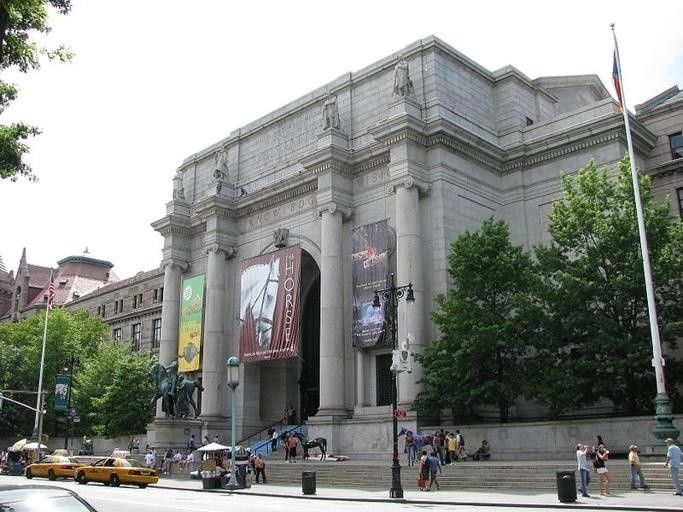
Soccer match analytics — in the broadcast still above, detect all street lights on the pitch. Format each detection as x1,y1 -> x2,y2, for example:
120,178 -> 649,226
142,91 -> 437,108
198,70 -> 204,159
226,355 -> 241,490
370,272 -> 413,500
63,348 -> 85,447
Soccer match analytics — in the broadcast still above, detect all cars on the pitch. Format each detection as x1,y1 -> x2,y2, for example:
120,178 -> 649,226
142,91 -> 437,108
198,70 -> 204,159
24,447 -> 158,489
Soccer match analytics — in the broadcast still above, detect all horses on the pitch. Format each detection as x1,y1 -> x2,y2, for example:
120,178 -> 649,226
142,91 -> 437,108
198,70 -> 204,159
148,362 -> 204,418
398,427 -> 436,466
240,254 -> 281,349
55,382 -> 69,400
290,431 -> 327,461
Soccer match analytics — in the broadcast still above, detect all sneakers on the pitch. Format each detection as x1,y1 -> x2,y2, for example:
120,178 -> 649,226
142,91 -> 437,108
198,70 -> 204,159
578,484 -> 683,498
418,488 -> 441,491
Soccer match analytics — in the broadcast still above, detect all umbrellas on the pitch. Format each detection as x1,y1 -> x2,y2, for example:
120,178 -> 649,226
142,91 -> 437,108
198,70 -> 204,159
12,439 -> 26,451
25,443 -> 47,449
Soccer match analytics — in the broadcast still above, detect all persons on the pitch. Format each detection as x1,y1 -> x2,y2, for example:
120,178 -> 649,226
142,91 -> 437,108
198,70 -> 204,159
393,54 -> 409,99
284,407 -> 289,425
173,168 -> 183,201
664,438 -> 683,495
629,445 -> 648,489
286,406 -> 295,425
576,444 -> 591,497
211,146 -> 229,180
128,429 -> 298,484
161,359 -> 179,396
176,375 -> 190,418
593,444 -> 612,495
405,428 -> 489,490
593,435 -> 604,459
321,89 -> 340,130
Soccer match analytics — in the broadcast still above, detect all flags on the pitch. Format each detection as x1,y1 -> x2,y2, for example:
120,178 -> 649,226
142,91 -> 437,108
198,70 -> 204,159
612,41 -> 622,111
48,274 -> 57,311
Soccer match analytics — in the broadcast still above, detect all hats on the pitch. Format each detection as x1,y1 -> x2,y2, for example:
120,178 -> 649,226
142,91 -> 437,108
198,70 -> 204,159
629,445 -> 637,451
665,437 -> 674,443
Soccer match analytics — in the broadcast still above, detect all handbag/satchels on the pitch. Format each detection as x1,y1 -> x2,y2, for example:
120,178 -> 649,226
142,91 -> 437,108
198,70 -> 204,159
417,479 -> 426,487
592,458 -> 606,469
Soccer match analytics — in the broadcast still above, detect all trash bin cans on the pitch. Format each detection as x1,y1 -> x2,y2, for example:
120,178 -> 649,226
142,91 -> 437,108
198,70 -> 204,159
302,471 -> 316,494
556,469 -> 577,503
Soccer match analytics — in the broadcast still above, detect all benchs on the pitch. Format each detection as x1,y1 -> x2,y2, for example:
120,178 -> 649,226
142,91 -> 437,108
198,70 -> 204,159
465,452 -> 491,461
589,449 -> 640,460
